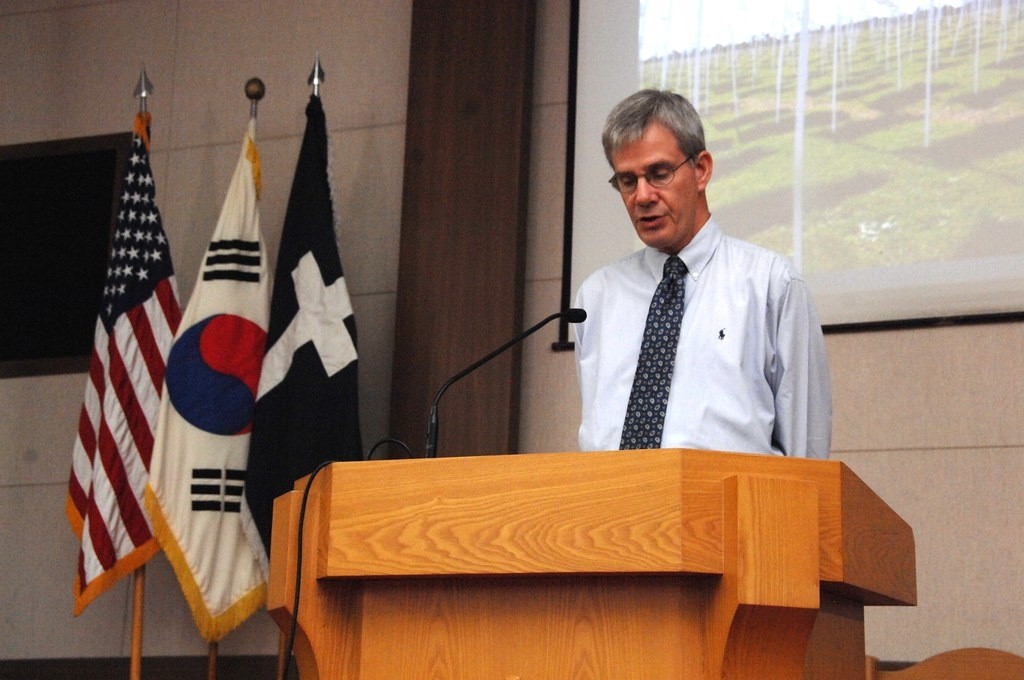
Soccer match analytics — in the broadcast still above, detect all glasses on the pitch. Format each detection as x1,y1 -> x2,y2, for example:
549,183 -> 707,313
609,155 -> 692,193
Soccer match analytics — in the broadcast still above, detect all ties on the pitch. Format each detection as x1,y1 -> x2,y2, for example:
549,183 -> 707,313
618,256 -> 688,450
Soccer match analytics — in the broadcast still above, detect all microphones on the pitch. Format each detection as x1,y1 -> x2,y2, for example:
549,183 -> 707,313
423,308 -> 587,458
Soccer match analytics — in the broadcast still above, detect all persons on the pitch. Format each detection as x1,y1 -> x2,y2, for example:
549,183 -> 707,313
570,90 -> 831,462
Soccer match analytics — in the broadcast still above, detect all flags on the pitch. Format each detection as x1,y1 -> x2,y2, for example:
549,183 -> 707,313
59,110 -> 182,620
141,116 -> 273,641
241,93 -> 365,583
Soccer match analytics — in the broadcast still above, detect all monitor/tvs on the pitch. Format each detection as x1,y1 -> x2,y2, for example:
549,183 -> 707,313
0,132 -> 132,378
